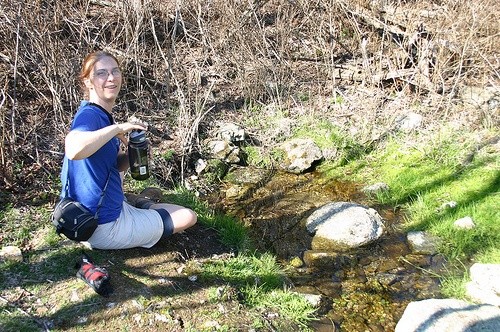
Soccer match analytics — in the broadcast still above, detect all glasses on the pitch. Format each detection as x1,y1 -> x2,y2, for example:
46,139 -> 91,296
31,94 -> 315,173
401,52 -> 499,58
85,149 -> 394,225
86,69 -> 122,79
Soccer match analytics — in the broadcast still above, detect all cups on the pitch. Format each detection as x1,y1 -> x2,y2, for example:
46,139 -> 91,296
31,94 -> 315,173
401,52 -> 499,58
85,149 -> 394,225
129,131 -> 149,181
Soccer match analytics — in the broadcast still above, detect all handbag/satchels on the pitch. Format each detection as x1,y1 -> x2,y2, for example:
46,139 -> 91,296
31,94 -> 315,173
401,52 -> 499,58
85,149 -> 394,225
51,198 -> 97,243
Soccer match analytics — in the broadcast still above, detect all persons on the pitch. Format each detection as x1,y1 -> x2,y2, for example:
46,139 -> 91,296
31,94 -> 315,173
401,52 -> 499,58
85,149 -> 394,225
61,51 -> 198,250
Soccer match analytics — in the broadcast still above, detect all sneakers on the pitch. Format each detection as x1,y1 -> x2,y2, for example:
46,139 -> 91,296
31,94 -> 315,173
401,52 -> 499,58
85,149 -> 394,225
124,186 -> 162,208
